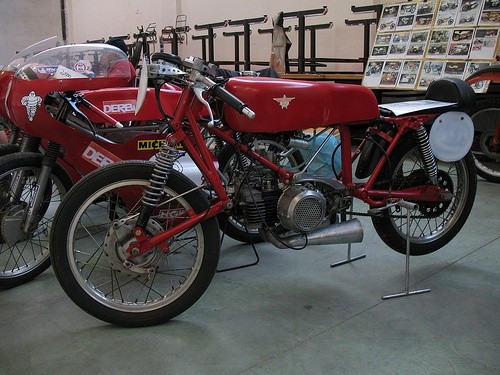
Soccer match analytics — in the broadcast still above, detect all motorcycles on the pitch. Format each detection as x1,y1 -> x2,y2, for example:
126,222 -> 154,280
49,57 -> 477,328
0,35 -> 307,290
366,0 -> 500,89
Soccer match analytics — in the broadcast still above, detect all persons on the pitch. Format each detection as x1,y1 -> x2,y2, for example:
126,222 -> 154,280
100,38 -> 136,78
0,116 -> 13,143
